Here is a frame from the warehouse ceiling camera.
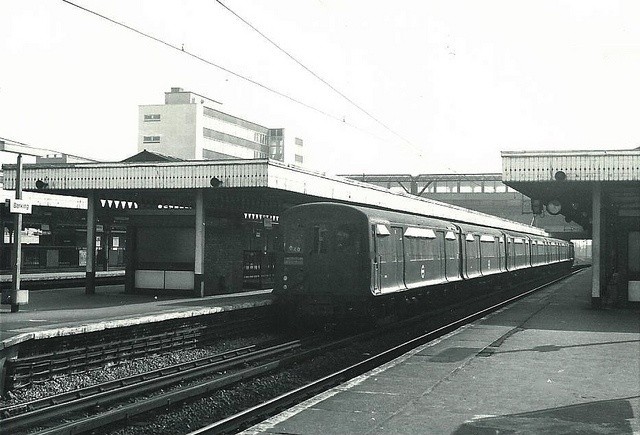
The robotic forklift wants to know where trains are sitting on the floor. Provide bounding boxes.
[271,201,575,324]
